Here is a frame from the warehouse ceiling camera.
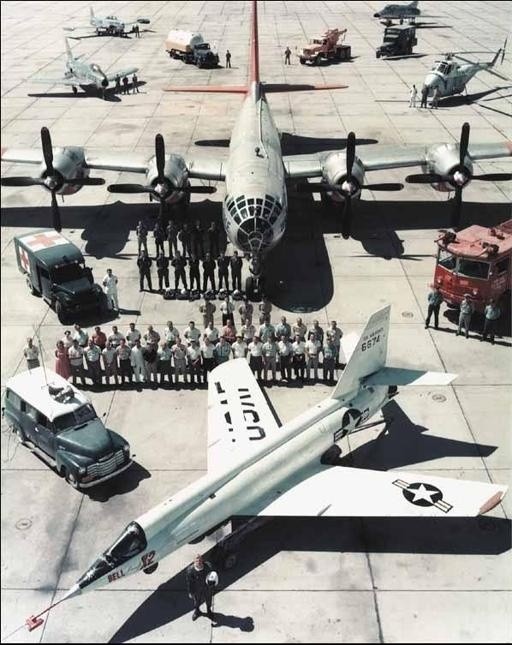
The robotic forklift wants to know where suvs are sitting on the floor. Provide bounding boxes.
[6,367,133,490]
[13,229,107,325]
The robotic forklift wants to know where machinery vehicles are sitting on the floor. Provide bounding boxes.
[298,28,346,66]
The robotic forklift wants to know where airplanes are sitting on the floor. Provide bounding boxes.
[3,303,510,640]
[2,2,512,285]
[24,37,141,99]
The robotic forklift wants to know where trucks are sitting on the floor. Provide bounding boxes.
[166,30,217,68]
[433,219,512,319]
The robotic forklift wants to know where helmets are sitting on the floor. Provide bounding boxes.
[204,570,221,587]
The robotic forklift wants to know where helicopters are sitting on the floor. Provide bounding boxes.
[373,1,451,28]
[374,39,507,103]
[64,3,153,40]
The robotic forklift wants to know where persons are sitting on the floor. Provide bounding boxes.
[409,85,417,108]
[238,298,253,327]
[132,74,139,93]
[116,339,133,385]
[276,335,293,384]
[186,553,214,620]
[260,296,272,327]
[184,321,201,346]
[74,323,89,346]
[142,341,158,388]
[136,221,148,257]
[292,318,307,342]
[291,334,306,382]
[97,25,140,39]
[231,337,248,359]
[431,85,440,109]
[154,222,165,258]
[123,75,129,94]
[166,220,178,260]
[200,337,217,384]
[305,334,322,381]
[189,253,200,293]
[114,74,121,95]
[137,251,154,292]
[62,332,74,349]
[157,342,173,387]
[24,338,40,370]
[247,337,264,384]
[173,338,188,387]
[84,342,101,388]
[261,338,280,385]
[217,251,230,293]
[200,296,216,330]
[226,50,231,67]
[91,328,106,349]
[215,338,231,364]
[164,322,179,348]
[222,319,236,344]
[284,47,291,65]
[480,298,501,343]
[143,326,161,352]
[156,252,169,292]
[101,341,119,388]
[192,220,206,260]
[126,323,141,347]
[103,269,120,313]
[131,340,146,385]
[68,340,87,387]
[308,321,323,367]
[325,321,343,367]
[241,319,256,344]
[420,85,429,108]
[220,297,235,326]
[322,336,338,386]
[55,340,70,380]
[425,285,443,330]
[179,223,192,258]
[457,294,475,338]
[275,317,292,341]
[207,223,221,260]
[258,318,275,343]
[231,252,243,293]
[203,322,219,345]
[185,340,202,387]
[108,327,126,347]
[171,251,188,291]
[202,253,216,293]
